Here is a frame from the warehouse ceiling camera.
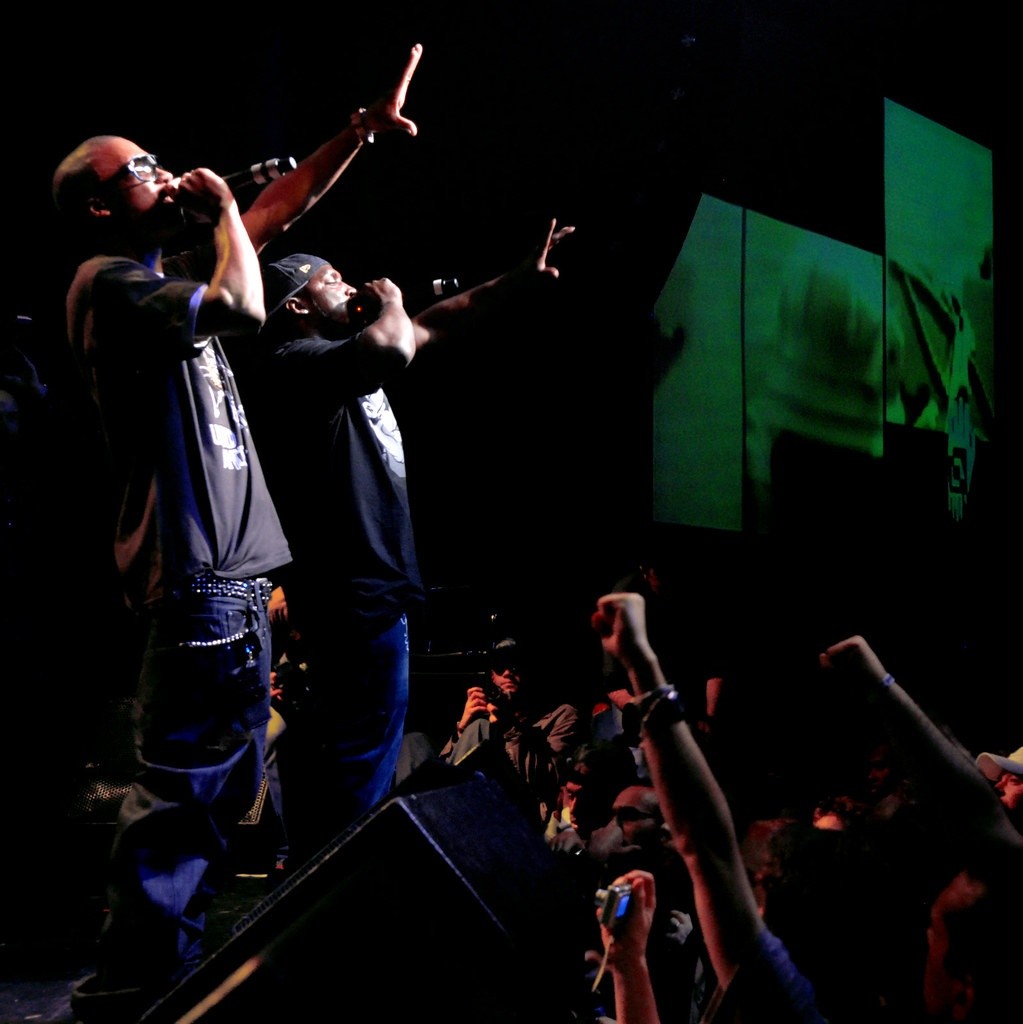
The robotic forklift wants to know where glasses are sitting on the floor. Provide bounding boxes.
[490,660,524,674]
[95,155,158,195]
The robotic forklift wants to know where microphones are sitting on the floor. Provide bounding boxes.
[221,157,296,190]
[401,279,460,303]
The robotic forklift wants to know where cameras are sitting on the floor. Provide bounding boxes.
[593,883,633,928]
[483,684,509,708]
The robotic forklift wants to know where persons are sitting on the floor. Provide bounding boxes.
[52,44,432,1024]
[257,219,594,880]
[585,589,1023,1024]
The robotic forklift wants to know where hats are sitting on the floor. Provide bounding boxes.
[974,745,1023,779]
[492,639,522,653]
[259,253,328,337]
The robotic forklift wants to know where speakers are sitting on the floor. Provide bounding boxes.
[138,774,598,1024]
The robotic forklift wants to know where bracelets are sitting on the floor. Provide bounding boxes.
[857,673,898,707]
[350,108,375,146]
[556,823,578,834]
[456,722,464,734]
[621,684,687,747]
